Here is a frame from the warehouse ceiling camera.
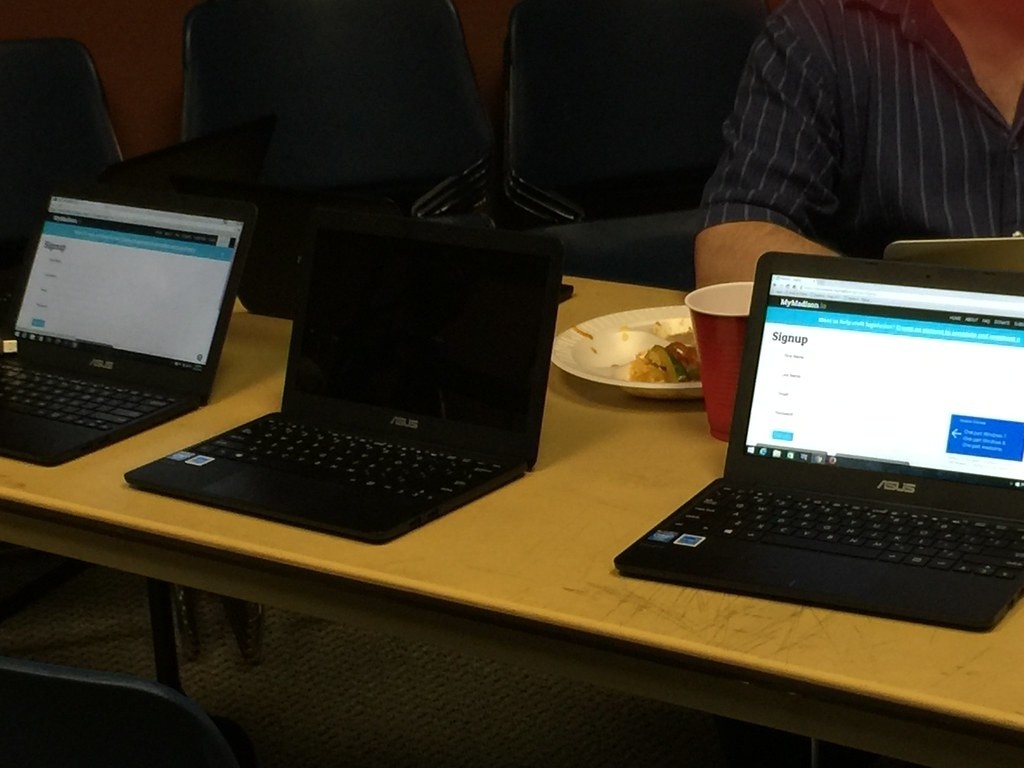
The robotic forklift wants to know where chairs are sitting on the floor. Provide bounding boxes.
[0,30,132,303]
[0,662,264,768]
[179,2,493,235]
[510,2,770,300]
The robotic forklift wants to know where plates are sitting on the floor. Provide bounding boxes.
[551,305,704,400]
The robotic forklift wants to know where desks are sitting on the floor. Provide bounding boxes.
[1,268,1016,733]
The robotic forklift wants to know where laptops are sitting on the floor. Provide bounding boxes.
[0,176,257,467]
[614,237,1024,634]
[128,207,567,547]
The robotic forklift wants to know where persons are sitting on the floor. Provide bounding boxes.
[695,0,1022,295]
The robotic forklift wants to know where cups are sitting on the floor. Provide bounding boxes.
[684,282,755,442]
